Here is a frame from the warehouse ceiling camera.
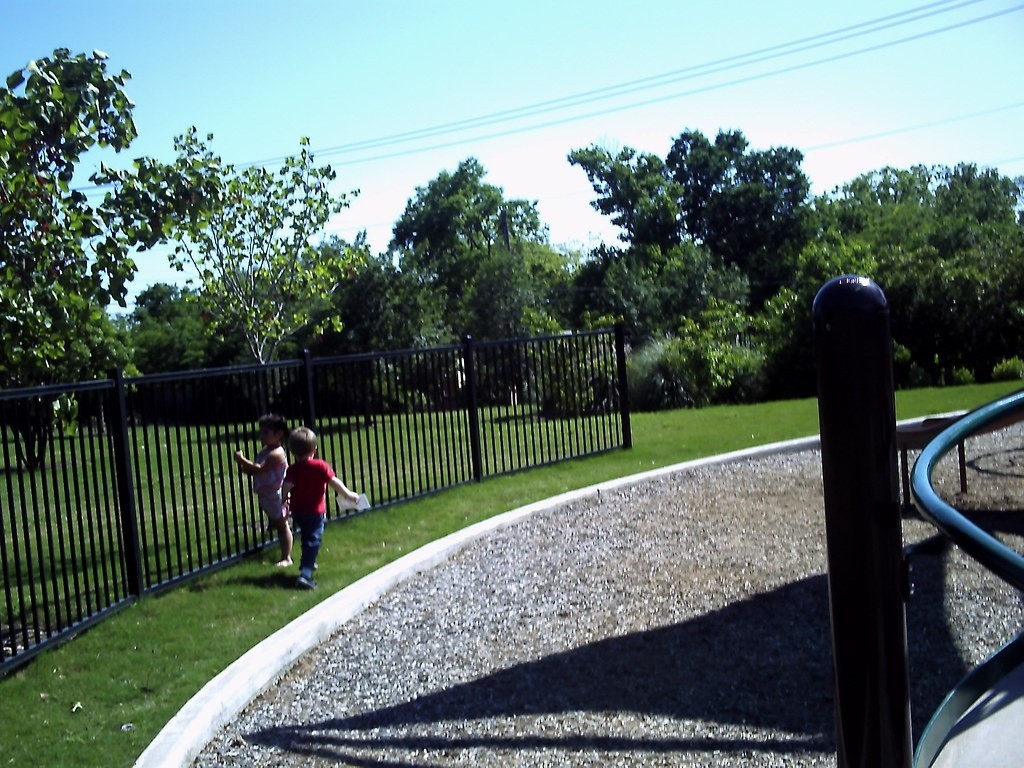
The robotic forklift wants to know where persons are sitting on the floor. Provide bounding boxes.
[234,415,295,568]
[280,426,360,589]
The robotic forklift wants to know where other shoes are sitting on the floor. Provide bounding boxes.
[298,564,318,570]
[275,560,293,568]
[298,575,315,589]
